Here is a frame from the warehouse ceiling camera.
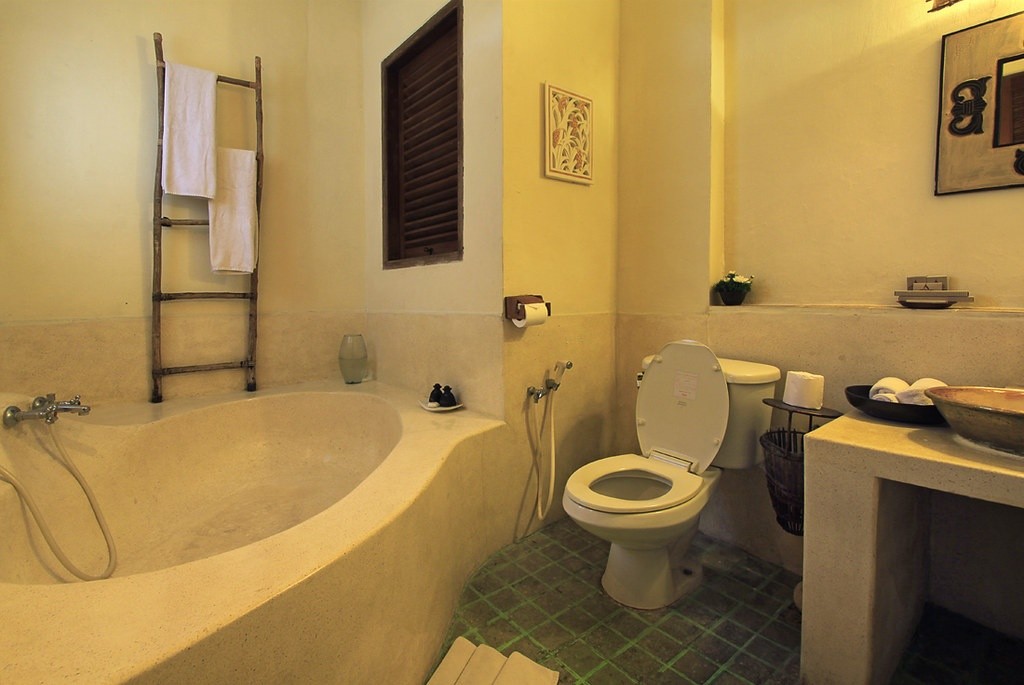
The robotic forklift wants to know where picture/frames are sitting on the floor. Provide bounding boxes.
[933,10,1024,196]
[546,80,596,186]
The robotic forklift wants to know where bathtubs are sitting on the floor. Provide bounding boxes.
[0,388,407,590]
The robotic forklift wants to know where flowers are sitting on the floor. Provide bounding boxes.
[714,269,754,293]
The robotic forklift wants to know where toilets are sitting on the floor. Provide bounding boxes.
[560,339,780,608]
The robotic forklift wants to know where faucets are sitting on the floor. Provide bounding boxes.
[31,394,91,419]
[3,392,58,427]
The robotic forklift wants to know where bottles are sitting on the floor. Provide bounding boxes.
[336,332,368,386]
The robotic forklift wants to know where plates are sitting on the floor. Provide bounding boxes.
[420,400,464,412]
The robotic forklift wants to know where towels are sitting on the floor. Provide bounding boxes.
[899,378,948,405]
[162,61,218,200]
[208,147,259,275]
[872,392,899,404]
[870,376,908,395]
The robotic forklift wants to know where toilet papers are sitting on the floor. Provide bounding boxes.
[509,304,548,328]
[785,371,825,410]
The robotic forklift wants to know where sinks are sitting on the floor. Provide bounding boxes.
[924,385,1024,457]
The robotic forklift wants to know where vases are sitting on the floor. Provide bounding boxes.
[720,289,746,306]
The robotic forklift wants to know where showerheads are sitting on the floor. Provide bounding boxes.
[525,361,576,519]
